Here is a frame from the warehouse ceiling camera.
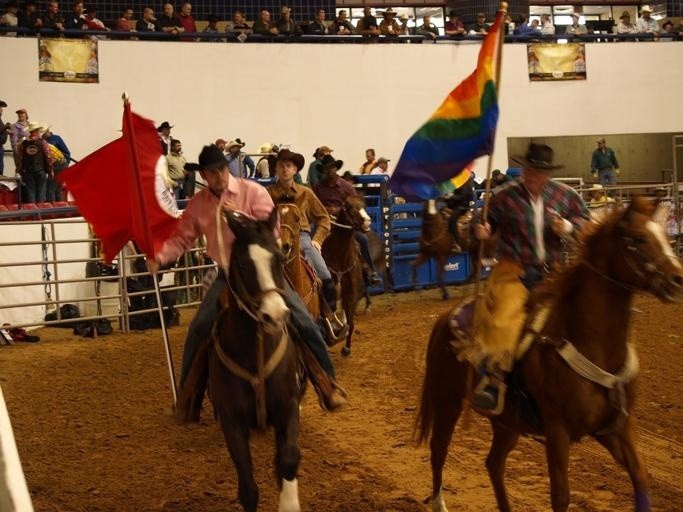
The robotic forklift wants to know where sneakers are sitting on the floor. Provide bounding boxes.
[471,383,498,408]
[323,390,348,410]
[450,244,462,251]
[369,271,383,286]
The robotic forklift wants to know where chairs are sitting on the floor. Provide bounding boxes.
[0,192,77,221]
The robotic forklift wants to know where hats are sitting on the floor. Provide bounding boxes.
[378,158,390,164]
[509,144,565,169]
[183,138,343,175]
[360,6,580,18]
[0,100,49,135]
[620,5,673,29]
[596,137,605,144]
[157,122,175,132]
[280,7,292,14]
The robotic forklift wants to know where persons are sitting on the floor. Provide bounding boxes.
[157,122,278,209]
[459,143,590,413]
[145,144,347,417]
[308,146,390,188]
[1,100,70,203]
[311,154,382,284]
[469,169,513,200]
[446,171,475,251]
[265,148,337,318]
[591,137,618,198]
[590,184,614,202]
[1,1,682,43]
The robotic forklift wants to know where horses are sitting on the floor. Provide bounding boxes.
[206,205,307,512]
[269,190,320,412]
[411,193,683,512]
[410,199,482,301]
[321,191,385,357]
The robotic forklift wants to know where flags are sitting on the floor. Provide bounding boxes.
[55,111,179,265]
[386,15,504,202]
